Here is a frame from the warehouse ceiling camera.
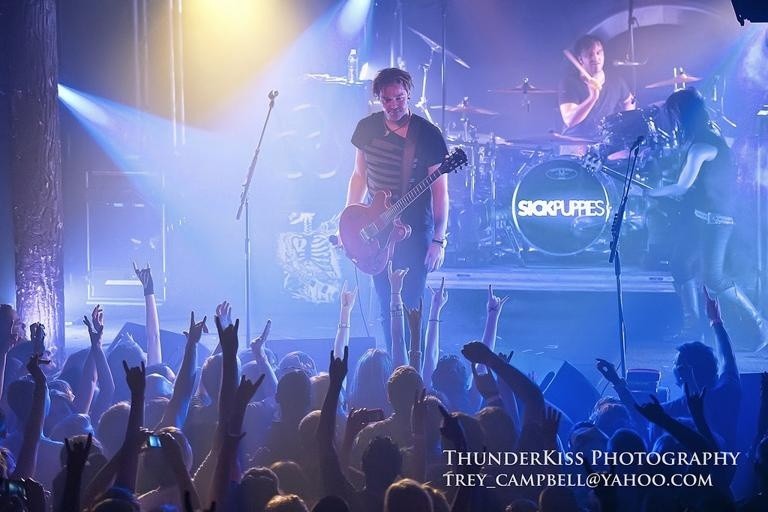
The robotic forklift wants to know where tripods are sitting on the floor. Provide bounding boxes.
[470,151,525,271]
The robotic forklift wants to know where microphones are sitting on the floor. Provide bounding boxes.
[631,135,647,151]
[268,88,280,100]
[613,60,645,70]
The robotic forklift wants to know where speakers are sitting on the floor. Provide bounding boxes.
[253,336,376,388]
[83,166,170,309]
[106,322,216,391]
[538,359,603,426]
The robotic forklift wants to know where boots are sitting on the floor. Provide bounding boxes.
[668,276,707,348]
[715,280,768,355]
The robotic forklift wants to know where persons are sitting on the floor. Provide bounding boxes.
[0,258,768,512]
[623,84,767,357]
[328,67,450,359]
[554,33,638,165]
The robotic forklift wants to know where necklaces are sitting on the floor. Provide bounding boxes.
[384,108,411,139]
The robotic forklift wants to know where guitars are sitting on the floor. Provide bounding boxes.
[580,153,688,214]
[339,147,469,276]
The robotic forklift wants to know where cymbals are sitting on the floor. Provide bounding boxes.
[504,133,597,145]
[448,104,498,115]
[647,100,666,109]
[487,83,567,95]
[645,74,704,90]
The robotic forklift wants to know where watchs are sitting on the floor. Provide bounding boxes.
[431,238,448,248]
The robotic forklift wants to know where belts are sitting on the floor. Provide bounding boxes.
[693,207,734,226]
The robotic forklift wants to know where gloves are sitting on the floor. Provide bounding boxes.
[134,267,155,297]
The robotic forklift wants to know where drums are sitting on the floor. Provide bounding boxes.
[597,107,658,160]
[446,131,505,193]
[512,155,613,257]
